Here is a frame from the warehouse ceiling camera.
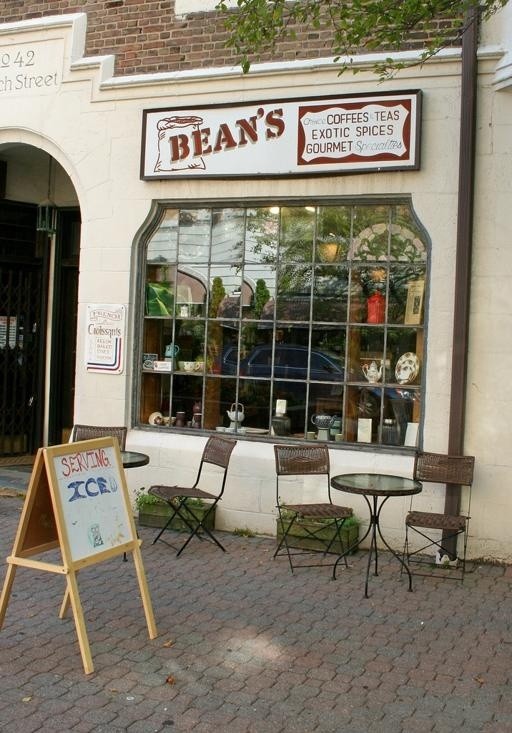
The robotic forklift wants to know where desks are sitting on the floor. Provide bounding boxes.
[328,472,422,601]
[115,442,149,564]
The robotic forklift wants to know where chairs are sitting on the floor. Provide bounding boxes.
[69,423,128,451]
[401,451,476,586]
[151,433,238,556]
[272,444,350,577]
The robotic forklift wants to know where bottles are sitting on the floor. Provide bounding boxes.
[383,418,397,444]
[367,288,385,325]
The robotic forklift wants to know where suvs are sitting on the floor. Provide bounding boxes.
[221,345,397,419]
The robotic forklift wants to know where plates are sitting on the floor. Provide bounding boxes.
[246,427,270,434]
[148,412,163,426]
[394,351,420,385]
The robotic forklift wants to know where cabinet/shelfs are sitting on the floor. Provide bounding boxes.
[140,264,426,447]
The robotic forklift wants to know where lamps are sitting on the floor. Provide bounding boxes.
[36,154,58,240]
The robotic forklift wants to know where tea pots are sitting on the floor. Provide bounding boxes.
[226,402,245,422]
[361,360,383,383]
[164,343,180,357]
[310,412,338,429]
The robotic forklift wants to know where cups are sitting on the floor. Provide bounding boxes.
[306,431,316,439]
[165,412,202,428]
[181,307,189,318]
[216,427,246,435]
[178,361,204,372]
[334,434,345,442]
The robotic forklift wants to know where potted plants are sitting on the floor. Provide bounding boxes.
[276,503,362,557]
[134,488,216,536]
